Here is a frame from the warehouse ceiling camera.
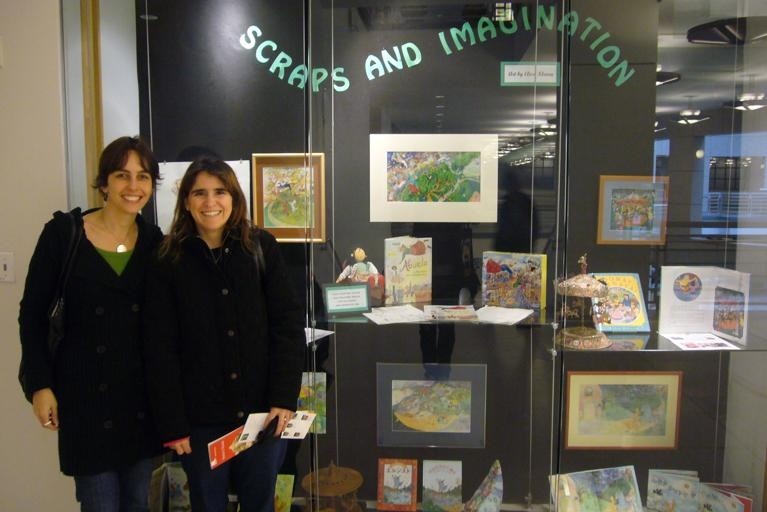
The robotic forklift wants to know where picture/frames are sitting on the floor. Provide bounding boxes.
[250,152,325,245]
[563,369,683,452]
[422,460,462,506]
[377,459,418,512]
[367,131,499,226]
[373,360,487,452]
[597,175,670,248]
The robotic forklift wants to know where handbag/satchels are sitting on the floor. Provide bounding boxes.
[43,209,85,380]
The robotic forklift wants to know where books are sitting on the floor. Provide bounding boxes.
[383,235,432,303]
[645,468,700,512]
[701,482,756,512]
[478,251,546,310]
[588,271,651,334]
[658,265,751,346]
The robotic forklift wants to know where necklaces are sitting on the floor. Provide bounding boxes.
[102,209,137,254]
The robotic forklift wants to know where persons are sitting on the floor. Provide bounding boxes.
[412,153,469,390]
[136,158,307,510]
[489,154,541,311]
[16,133,165,512]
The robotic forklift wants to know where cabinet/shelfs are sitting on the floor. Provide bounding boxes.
[309,0,564,511]
[551,1,767,512]
[83,0,317,512]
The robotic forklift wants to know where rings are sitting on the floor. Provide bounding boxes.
[43,420,52,427]
